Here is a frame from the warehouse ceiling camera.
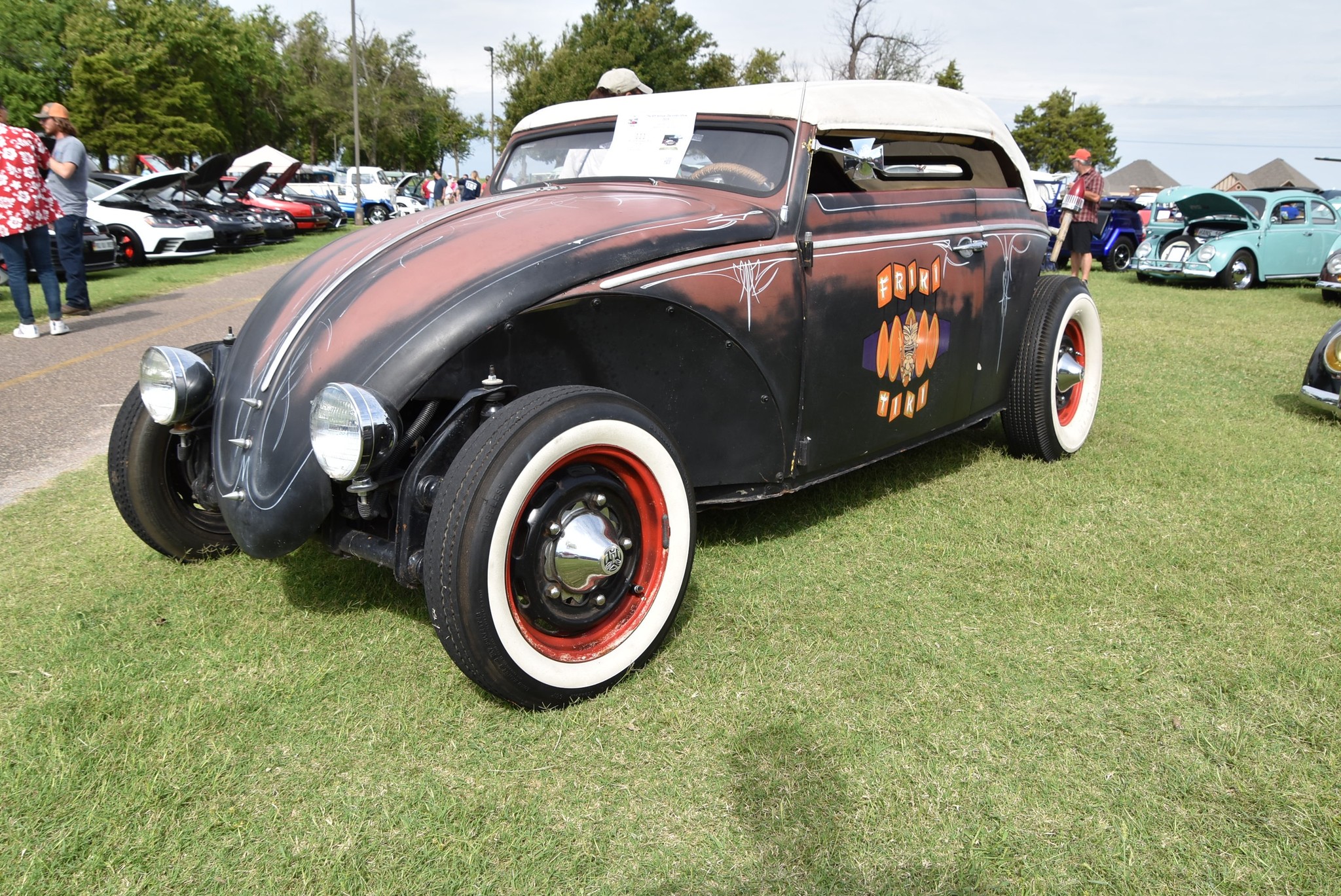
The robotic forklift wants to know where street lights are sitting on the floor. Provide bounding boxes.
[484,45,494,173]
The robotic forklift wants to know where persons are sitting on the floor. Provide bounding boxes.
[420,169,490,210]
[38,104,91,315]
[501,173,543,191]
[588,66,653,100]
[1280,202,1300,220]
[0,102,71,338]
[1059,148,1104,283]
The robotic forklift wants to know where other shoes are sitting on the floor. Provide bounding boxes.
[49,320,69,335]
[13,323,40,338]
[61,304,89,316]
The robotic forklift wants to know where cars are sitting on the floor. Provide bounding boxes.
[1033,176,1341,420]
[0,154,428,283]
[106,78,1101,711]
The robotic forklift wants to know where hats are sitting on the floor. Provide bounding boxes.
[597,67,653,94]
[1068,149,1091,161]
[31,102,70,119]
[448,173,452,176]
